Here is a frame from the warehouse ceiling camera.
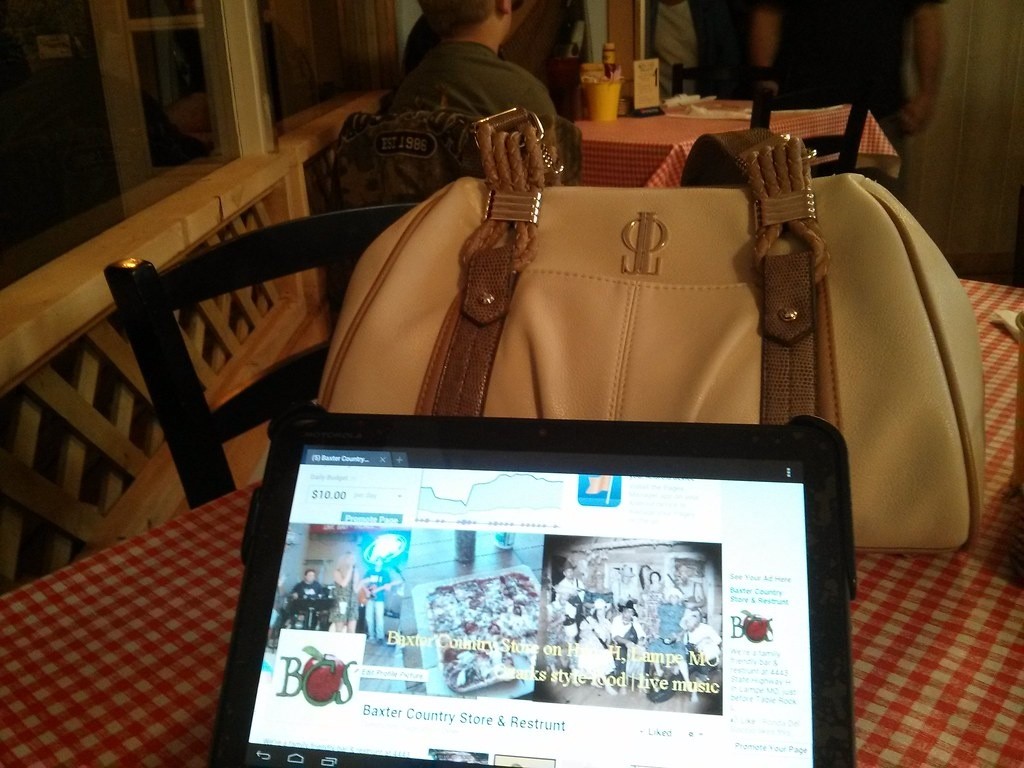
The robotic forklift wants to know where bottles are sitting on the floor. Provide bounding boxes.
[603,42,616,79]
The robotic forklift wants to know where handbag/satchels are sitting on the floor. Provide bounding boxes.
[314,108,986,557]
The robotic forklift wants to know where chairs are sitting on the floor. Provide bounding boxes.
[752,78,871,178]
[102,203,421,512]
[327,107,583,226]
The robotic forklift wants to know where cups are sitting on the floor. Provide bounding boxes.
[455,530,476,562]
[584,80,622,122]
[495,531,516,549]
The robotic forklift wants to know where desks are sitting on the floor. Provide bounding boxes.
[0,276,1024,768]
[574,100,901,189]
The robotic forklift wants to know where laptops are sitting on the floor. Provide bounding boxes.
[217,410,855,768]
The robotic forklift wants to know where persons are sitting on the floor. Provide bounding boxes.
[546,564,722,712]
[650,0,949,194]
[390,0,558,187]
[287,556,393,644]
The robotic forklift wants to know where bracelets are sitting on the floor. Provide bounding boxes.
[748,66,778,84]
